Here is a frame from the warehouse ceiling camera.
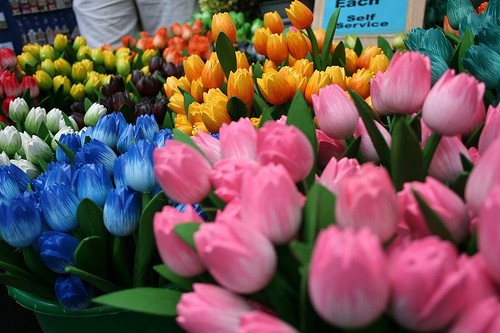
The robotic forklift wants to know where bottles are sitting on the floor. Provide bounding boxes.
[10,0,56,16]
[16,17,72,47]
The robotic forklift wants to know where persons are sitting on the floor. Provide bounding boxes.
[73,0,196,51]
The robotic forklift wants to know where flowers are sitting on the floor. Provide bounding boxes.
[0,0,500,333]
